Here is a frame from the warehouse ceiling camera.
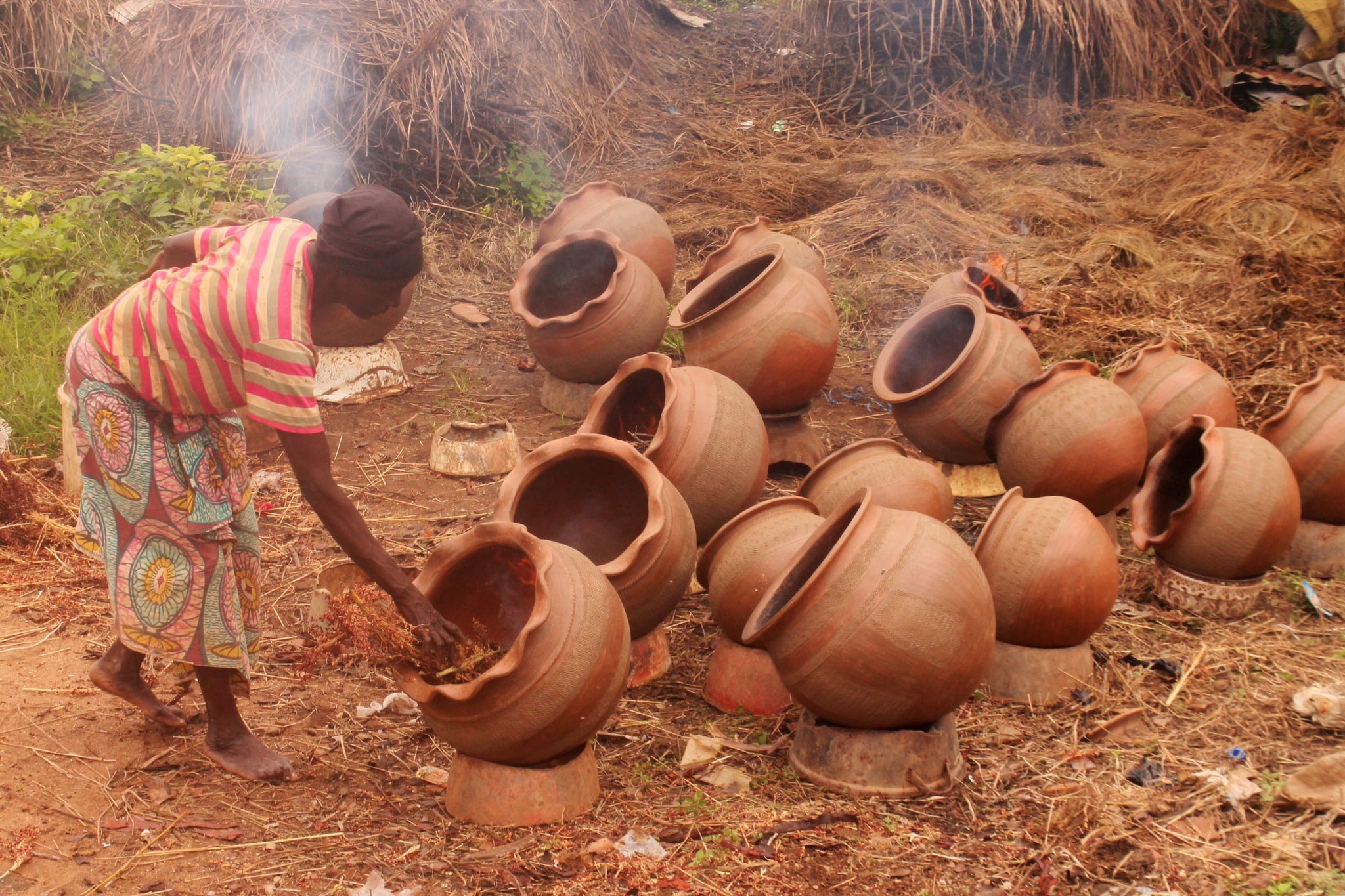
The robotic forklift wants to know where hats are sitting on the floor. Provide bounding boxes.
[317,184,426,280]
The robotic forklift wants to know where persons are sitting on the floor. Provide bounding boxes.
[52,176,466,784]
